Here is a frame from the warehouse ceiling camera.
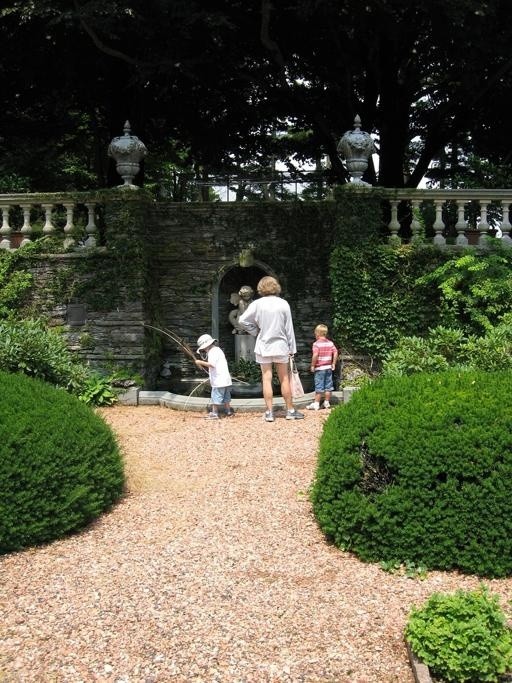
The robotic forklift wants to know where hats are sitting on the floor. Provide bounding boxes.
[196,333,219,355]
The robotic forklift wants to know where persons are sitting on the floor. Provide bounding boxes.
[238,285,258,333]
[228,291,243,332]
[193,332,235,422]
[305,321,339,410]
[236,274,306,421]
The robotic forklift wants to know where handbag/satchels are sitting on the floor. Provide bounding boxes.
[288,355,305,399]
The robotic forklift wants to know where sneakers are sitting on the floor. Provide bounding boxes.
[203,408,234,420]
[285,408,304,420]
[305,402,320,411]
[320,402,331,409]
[262,409,275,422]
